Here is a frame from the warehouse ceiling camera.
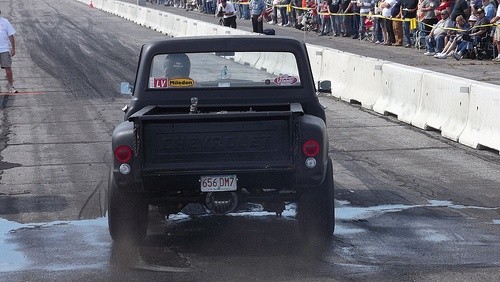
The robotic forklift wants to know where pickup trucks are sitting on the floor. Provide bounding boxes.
[107,34,336,249]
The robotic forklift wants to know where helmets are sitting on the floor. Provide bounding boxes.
[163,54,191,76]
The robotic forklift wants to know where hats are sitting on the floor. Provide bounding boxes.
[469,15,477,21]
[476,8,484,13]
[441,10,447,14]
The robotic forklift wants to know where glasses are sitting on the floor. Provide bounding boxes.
[477,13,481,14]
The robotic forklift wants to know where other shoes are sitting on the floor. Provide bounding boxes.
[492,56,500,62]
[334,33,340,37]
[405,44,410,48]
[424,51,434,55]
[434,52,439,57]
[352,34,358,39]
[447,51,454,57]
[452,52,460,60]
[8,87,18,93]
[375,41,402,46]
[438,52,447,59]
[343,34,350,37]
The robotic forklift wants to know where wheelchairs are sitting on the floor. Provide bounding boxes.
[462,28,498,60]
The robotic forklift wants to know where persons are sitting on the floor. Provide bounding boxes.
[235,0,250,20]
[266,0,500,62]
[147,0,217,15]
[215,0,237,29]
[250,0,266,34]
[163,52,201,88]
[0,10,19,93]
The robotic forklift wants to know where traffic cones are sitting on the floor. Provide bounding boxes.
[89,0,93,8]
